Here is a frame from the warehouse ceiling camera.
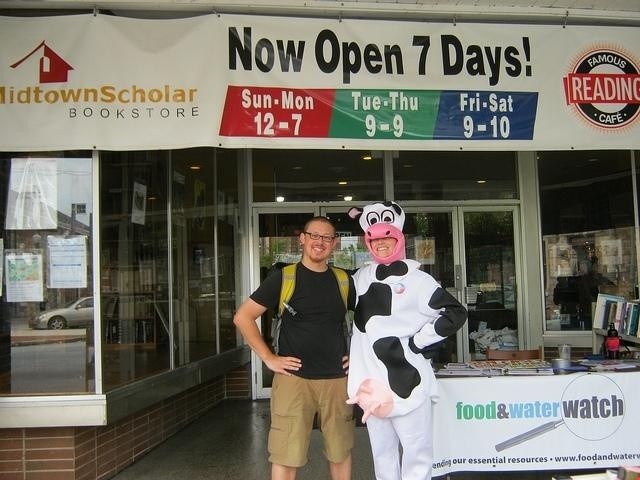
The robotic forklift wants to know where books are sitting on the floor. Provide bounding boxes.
[438,359,553,375]
[593,292,640,337]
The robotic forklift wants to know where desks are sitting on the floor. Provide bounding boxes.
[431,357,639,478]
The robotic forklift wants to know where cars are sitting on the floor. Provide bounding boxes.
[29,296,93,330]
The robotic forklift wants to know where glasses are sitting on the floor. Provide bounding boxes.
[304,231,335,242]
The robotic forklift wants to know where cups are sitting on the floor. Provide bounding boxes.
[559,344,571,365]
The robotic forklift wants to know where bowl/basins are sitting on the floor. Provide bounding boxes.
[550,357,570,369]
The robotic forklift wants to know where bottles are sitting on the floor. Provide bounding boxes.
[605,322,620,361]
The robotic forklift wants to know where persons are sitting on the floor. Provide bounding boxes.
[553,276,591,330]
[233,216,356,480]
[345,237,468,480]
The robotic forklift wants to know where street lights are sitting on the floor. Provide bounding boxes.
[33,232,46,312]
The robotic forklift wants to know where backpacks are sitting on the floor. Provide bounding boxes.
[271,264,352,354]
